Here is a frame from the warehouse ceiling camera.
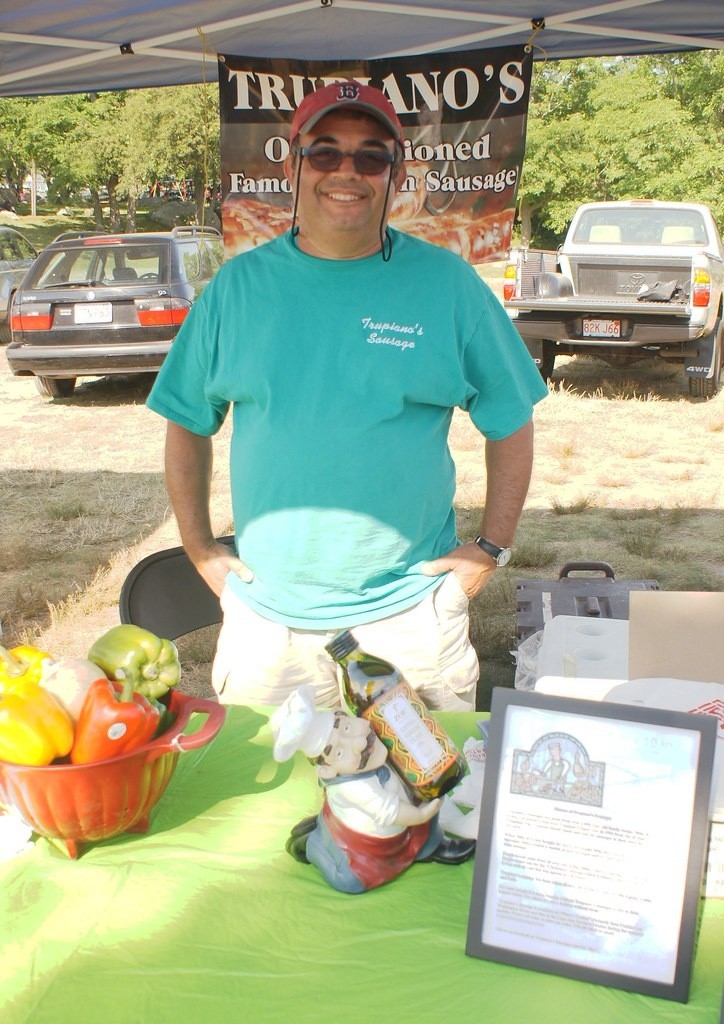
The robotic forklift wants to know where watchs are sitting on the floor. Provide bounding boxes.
[474,535,512,567]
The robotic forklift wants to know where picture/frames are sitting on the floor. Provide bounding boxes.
[463,688,718,1005]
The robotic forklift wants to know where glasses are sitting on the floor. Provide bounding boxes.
[300,145,395,176]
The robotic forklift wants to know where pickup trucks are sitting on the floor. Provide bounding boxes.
[503,198,724,396]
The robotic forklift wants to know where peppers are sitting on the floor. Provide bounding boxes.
[0,624,181,767]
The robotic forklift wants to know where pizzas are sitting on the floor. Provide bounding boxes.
[221,197,299,259]
[384,158,515,263]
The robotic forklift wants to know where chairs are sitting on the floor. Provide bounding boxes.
[112,266,139,282]
[118,534,237,642]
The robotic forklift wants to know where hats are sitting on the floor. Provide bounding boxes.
[289,81,405,159]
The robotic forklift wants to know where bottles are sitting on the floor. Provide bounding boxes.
[322,628,467,801]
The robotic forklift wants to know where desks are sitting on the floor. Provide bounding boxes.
[0,702,722,1024]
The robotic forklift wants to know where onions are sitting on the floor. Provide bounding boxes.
[39,657,108,722]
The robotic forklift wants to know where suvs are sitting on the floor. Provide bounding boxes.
[5,224,224,400]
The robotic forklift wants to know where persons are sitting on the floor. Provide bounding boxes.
[270,683,477,894]
[143,81,548,712]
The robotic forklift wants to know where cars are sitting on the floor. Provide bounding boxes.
[140,180,180,199]
[181,179,196,199]
[0,227,41,342]
[86,185,128,203]
[20,186,45,205]
[0,187,18,210]
